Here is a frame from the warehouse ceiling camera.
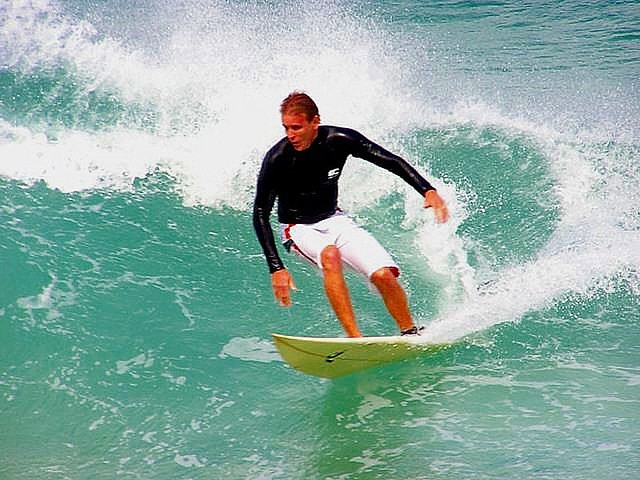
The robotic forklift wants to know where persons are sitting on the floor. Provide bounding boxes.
[252,90,448,336]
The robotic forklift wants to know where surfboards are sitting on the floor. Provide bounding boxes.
[271,333,455,378]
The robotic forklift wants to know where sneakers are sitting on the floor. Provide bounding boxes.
[401,326,424,336]
